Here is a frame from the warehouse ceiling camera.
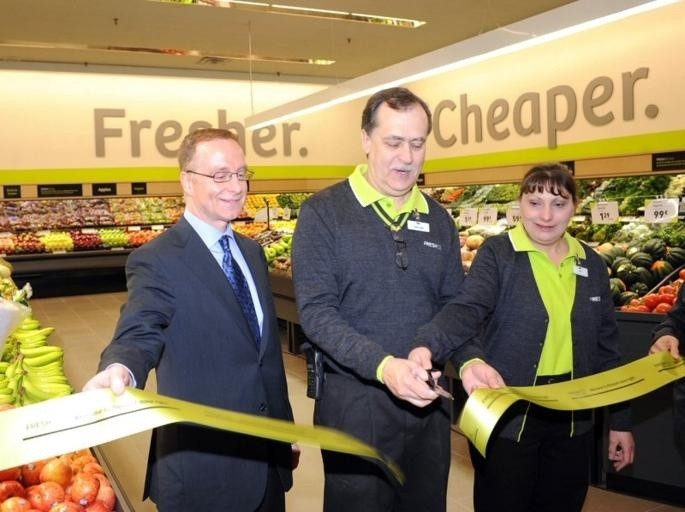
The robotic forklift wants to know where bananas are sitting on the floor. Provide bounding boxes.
[0,311,75,407]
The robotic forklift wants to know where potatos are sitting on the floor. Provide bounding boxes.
[460,235,485,272]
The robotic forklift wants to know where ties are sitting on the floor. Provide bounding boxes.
[217,237,262,350]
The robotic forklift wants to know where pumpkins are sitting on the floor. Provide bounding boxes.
[597,239,685,308]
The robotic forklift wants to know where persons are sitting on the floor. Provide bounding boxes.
[80,127,302,511]
[649,278,685,461]
[291,88,508,510]
[407,166,637,511]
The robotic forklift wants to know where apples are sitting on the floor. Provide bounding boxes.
[0,403,116,512]
[264,234,292,269]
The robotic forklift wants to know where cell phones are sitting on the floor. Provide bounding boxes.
[306,347,324,400]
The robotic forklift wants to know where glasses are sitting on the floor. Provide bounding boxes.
[185,168,255,184]
